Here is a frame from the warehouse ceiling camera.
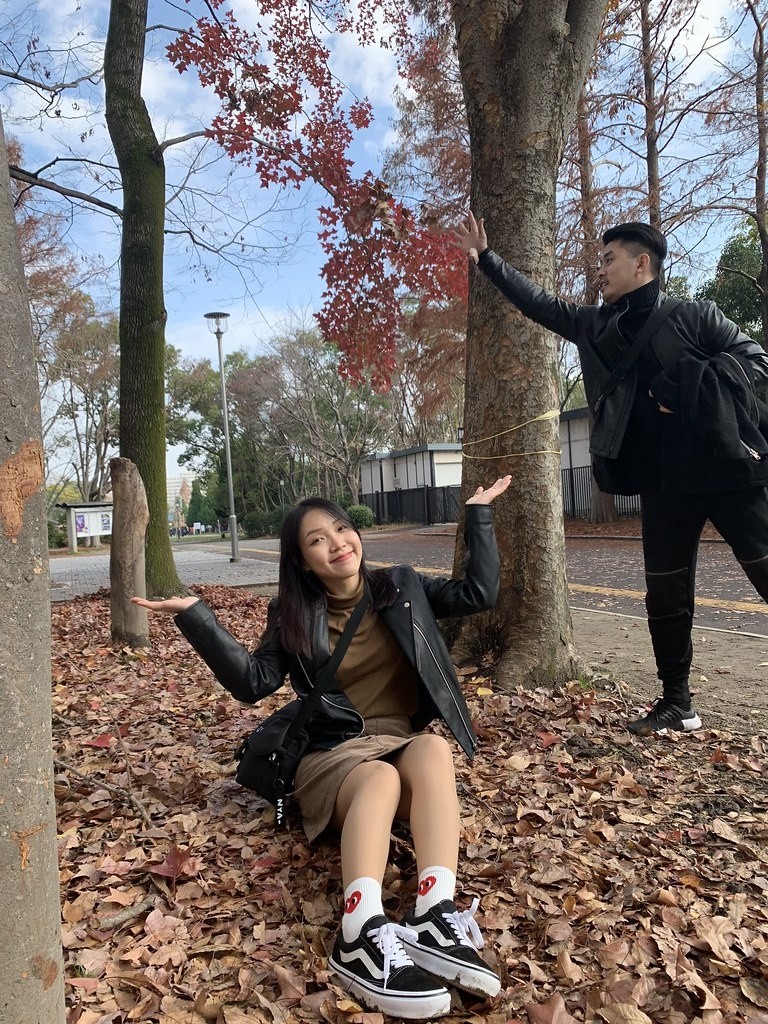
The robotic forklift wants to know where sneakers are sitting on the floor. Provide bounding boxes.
[397,898,501,998]
[628,697,703,738]
[328,914,452,1020]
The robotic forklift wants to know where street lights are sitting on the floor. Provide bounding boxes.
[204,311,239,562]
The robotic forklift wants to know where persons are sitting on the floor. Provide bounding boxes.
[448,210,768,736]
[130,475,512,1019]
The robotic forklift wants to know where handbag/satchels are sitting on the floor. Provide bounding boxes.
[233,697,317,831]
